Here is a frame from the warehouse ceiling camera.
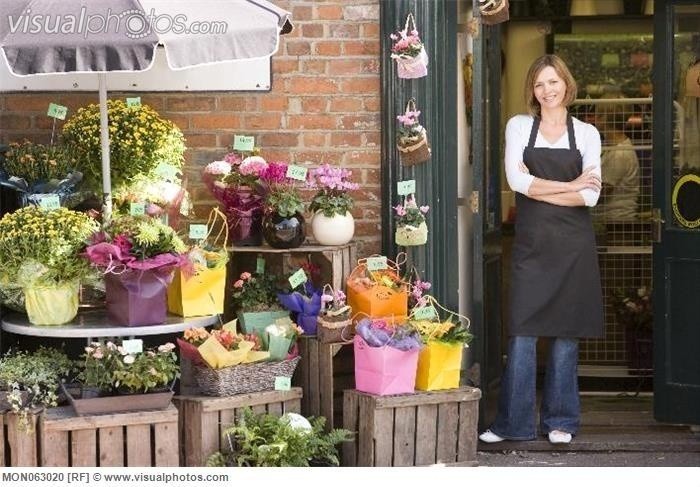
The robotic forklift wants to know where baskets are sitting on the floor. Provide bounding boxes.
[190,356,302,397]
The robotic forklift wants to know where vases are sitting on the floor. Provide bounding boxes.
[236,311,291,335]
[312,208,355,245]
[262,210,306,249]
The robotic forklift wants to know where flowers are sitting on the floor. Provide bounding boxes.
[234,273,285,312]
[354,319,420,351]
[396,110,425,146]
[204,151,270,201]
[279,264,328,300]
[320,283,347,312]
[80,214,190,269]
[350,265,407,294]
[394,198,430,230]
[181,240,229,269]
[259,316,305,362]
[183,318,270,369]
[4,139,87,195]
[304,163,361,217]
[63,100,188,203]
[0,207,102,290]
[410,314,474,344]
[389,30,422,60]
[411,280,432,308]
[73,341,181,394]
[259,162,304,217]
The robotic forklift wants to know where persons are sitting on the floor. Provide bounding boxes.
[476,54,603,446]
[621,66,685,194]
[589,90,642,268]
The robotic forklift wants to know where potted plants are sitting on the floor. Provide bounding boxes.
[206,407,358,467]
[1,345,72,436]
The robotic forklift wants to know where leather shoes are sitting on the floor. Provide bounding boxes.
[548,430,572,444]
[479,428,507,443]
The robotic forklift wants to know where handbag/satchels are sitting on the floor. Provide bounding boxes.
[316,284,351,344]
[395,185,428,247]
[396,13,429,79]
[165,208,229,316]
[480,0,510,26]
[408,296,471,392]
[396,100,432,167]
[96,243,179,327]
[348,252,408,334]
[342,312,420,396]
